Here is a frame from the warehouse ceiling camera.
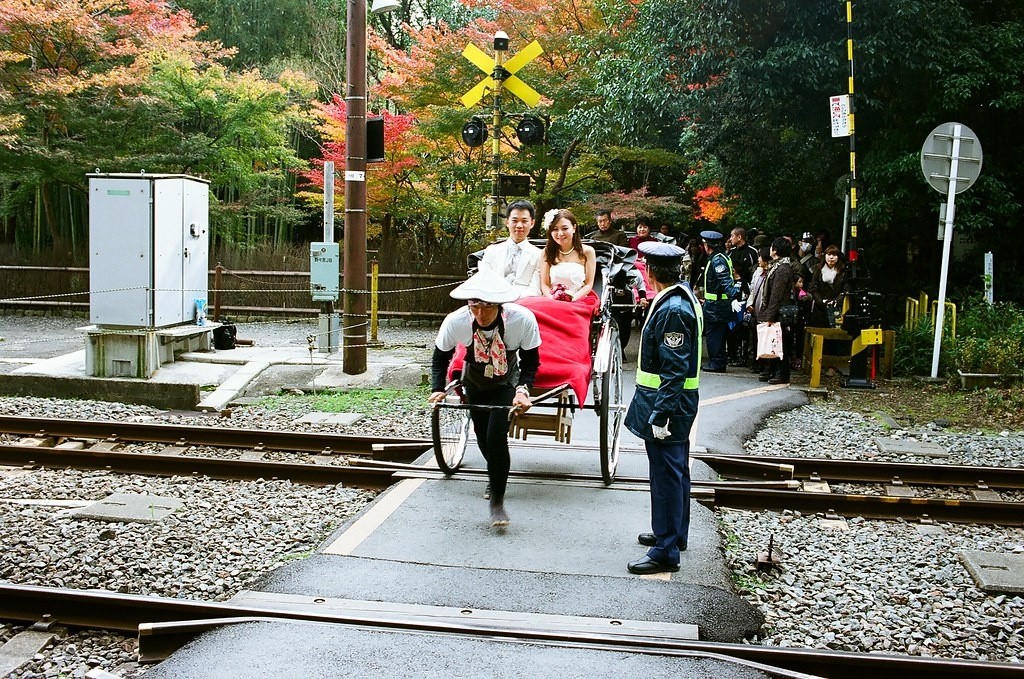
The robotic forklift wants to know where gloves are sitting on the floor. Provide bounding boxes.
[652,417,672,440]
[731,299,742,313]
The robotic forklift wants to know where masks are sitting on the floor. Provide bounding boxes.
[800,242,812,252]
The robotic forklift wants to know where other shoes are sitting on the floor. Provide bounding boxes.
[484,482,491,498]
[490,499,511,528]
[729,358,774,376]
[759,375,790,384]
[702,364,726,373]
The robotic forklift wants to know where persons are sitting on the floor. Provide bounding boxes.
[621,241,705,574]
[589,210,845,383]
[536,207,594,302]
[428,268,542,526]
[478,199,548,296]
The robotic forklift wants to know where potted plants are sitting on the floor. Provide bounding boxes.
[948,335,1024,390]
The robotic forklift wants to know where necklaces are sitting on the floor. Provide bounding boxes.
[558,245,574,255]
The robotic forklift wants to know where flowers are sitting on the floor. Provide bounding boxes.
[550,283,573,302]
[542,209,558,230]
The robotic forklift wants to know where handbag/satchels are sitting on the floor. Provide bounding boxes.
[779,305,799,324]
[756,322,783,360]
[744,312,756,324]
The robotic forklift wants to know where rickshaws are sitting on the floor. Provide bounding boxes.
[586,231,676,323]
[432,237,628,484]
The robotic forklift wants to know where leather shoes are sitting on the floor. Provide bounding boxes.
[627,554,682,575]
[638,532,687,551]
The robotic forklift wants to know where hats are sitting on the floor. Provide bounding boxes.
[637,241,685,265]
[798,232,815,244]
[449,269,521,304]
[701,231,723,242]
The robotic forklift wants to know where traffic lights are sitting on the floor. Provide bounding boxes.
[463,121,488,147]
[517,118,545,145]
[501,176,530,197]
